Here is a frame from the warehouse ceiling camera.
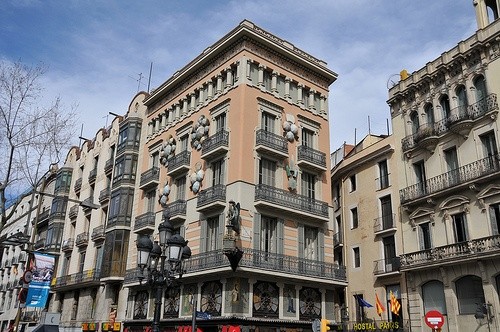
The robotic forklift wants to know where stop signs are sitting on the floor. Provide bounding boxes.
[424,310,444,330]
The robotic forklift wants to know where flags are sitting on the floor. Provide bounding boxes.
[390,290,401,316]
[375,293,386,317]
[25,251,56,309]
[357,294,374,308]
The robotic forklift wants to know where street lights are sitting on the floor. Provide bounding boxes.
[12,175,100,332]
[135,205,191,332]
